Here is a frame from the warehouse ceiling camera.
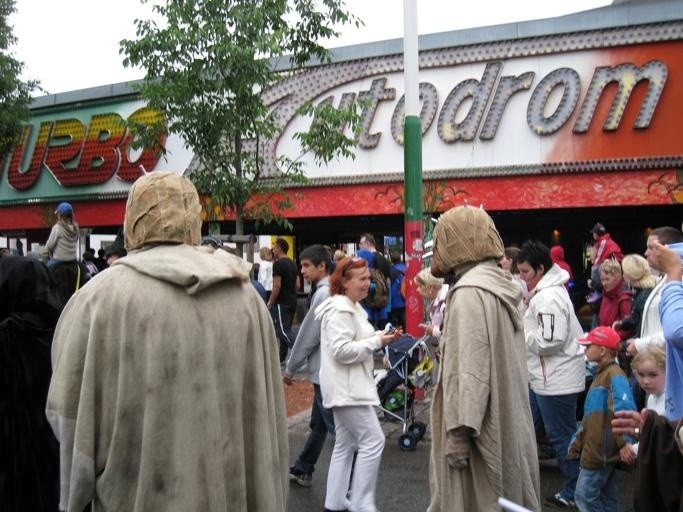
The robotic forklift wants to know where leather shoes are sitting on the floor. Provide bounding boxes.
[443,426,471,470]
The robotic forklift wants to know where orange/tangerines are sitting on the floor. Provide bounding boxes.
[373,320,433,453]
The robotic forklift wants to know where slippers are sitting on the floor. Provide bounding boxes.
[363,267,389,310]
[372,250,390,279]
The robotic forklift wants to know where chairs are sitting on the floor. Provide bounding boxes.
[279,361,286,370]
[537,446,558,463]
[289,464,313,487]
[543,492,578,510]
[295,356,308,373]
[586,290,602,304]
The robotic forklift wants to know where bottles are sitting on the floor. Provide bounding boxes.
[122,170,205,255]
[429,201,508,277]
[588,224,606,236]
[574,325,622,351]
[53,202,74,215]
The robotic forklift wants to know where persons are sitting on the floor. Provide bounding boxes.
[49,172,683,511]
